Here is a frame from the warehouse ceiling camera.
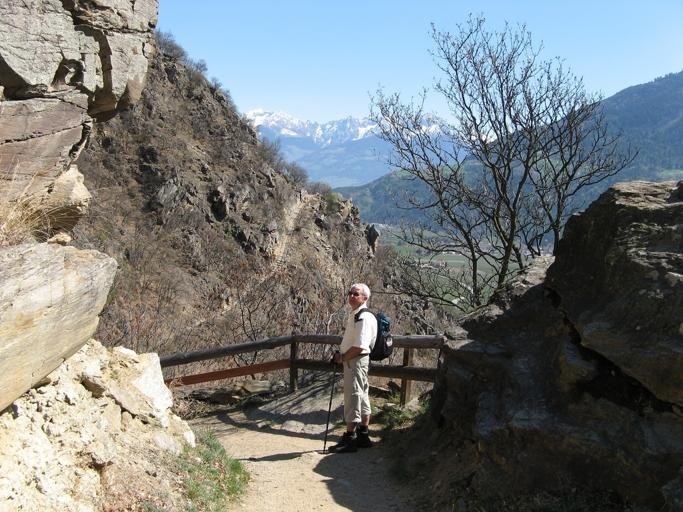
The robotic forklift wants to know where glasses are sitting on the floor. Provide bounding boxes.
[349,291,359,296]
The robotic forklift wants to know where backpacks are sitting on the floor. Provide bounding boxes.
[354,308,393,361]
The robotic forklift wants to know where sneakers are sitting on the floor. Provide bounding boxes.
[329,429,373,453]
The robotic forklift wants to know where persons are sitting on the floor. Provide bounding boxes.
[327,282,379,453]
